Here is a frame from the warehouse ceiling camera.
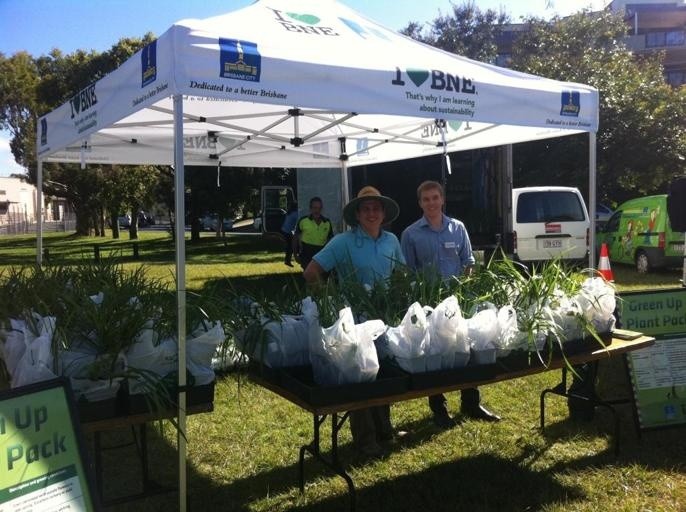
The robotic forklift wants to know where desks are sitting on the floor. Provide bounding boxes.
[76,372,214,506]
[248,311,656,512]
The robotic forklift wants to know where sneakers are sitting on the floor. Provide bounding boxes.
[285,261,294,267]
[360,435,406,461]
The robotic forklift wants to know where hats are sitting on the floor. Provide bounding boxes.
[343,186,401,226]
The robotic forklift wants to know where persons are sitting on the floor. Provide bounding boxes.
[280,201,297,268]
[302,187,406,456]
[292,196,334,272]
[401,181,501,427]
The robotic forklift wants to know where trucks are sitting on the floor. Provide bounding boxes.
[260,142,516,271]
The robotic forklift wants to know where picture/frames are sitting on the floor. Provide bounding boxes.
[614,287,685,433]
[0,377,102,512]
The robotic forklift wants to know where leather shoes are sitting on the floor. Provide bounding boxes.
[463,404,501,422]
[434,410,456,429]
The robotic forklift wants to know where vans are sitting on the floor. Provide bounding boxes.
[594,192,684,276]
[511,183,594,270]
